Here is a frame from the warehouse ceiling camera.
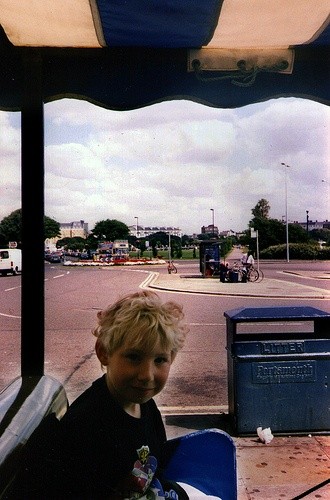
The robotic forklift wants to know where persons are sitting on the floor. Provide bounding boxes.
[0,291,190,500]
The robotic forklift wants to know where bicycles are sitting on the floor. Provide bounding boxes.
[225,267,264,283]
[166,260,177,274]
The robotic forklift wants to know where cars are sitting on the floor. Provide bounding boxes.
[50,255,62,263]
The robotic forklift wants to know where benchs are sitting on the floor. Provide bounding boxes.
[0,375,237,500]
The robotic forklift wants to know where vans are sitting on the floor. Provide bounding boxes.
[0,249,21,276]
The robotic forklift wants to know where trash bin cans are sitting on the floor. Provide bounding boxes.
[229,265,249,283]
[131,252,138,257]
[318,242,327,249]
[143,251,153,260]
[223,304,330,438]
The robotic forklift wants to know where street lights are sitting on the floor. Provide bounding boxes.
[281,162,291,263]
[134,216,139,231]
[209,208,215,233]
